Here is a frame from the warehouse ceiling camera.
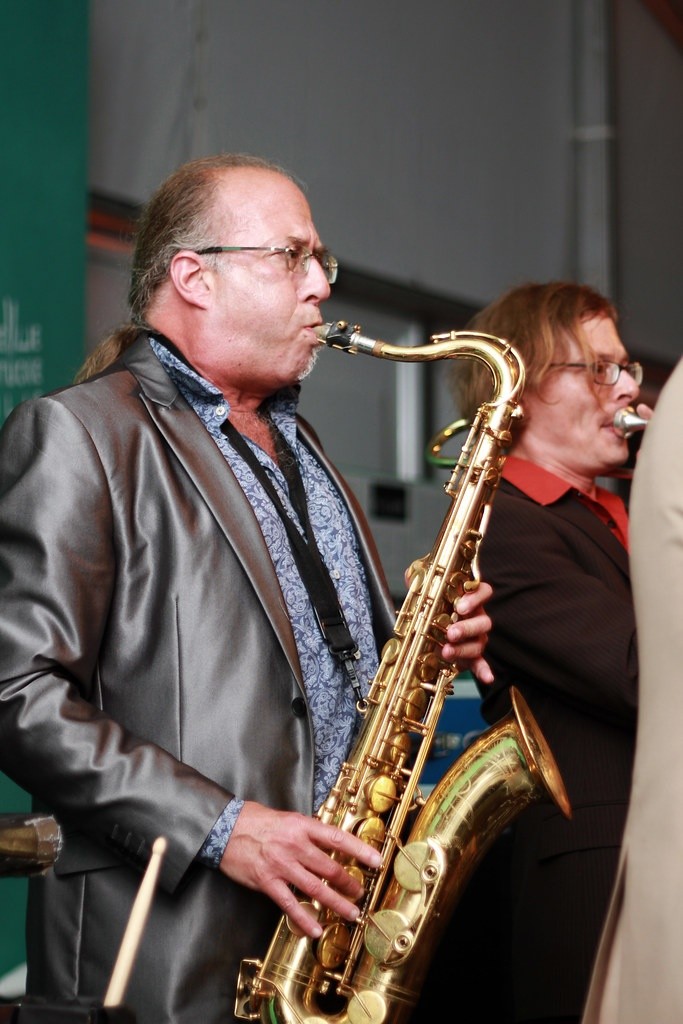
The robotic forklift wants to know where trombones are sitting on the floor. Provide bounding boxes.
[423,406,650,472]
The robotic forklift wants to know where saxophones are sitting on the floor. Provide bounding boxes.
[233,314,576,1024]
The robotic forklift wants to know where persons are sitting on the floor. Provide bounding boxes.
[444,278,683,1024]
[1,153,498,1024]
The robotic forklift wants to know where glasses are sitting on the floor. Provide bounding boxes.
[542,360,643,386]
[196,246,340,285]
